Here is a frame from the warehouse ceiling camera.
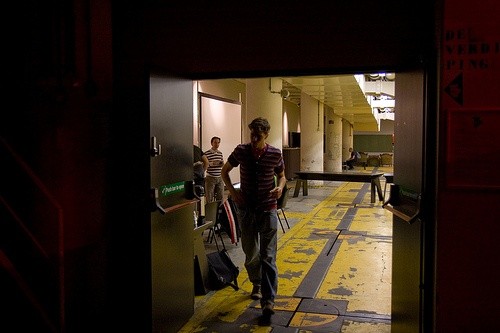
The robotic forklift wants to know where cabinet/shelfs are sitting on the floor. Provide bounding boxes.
[283,146,302,180]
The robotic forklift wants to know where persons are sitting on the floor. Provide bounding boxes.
[346,148,358,170]
[204,137,224,206]
[193,144,209,172]
[221,117,286,313]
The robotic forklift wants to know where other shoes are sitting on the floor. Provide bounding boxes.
[262,300,276,315]
[251,286,263,300]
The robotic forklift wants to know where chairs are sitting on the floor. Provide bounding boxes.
[277,182,290,233]
[378,153,394,167]
[204,196,240,250]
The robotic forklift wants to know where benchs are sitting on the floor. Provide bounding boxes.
[342,161,368,170]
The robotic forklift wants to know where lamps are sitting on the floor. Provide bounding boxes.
[279,88,291,101]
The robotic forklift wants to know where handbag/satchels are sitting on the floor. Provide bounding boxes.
[204,249,240,291]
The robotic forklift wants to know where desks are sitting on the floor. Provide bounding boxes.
[383,173,394,207]
[292,170,384,204]
